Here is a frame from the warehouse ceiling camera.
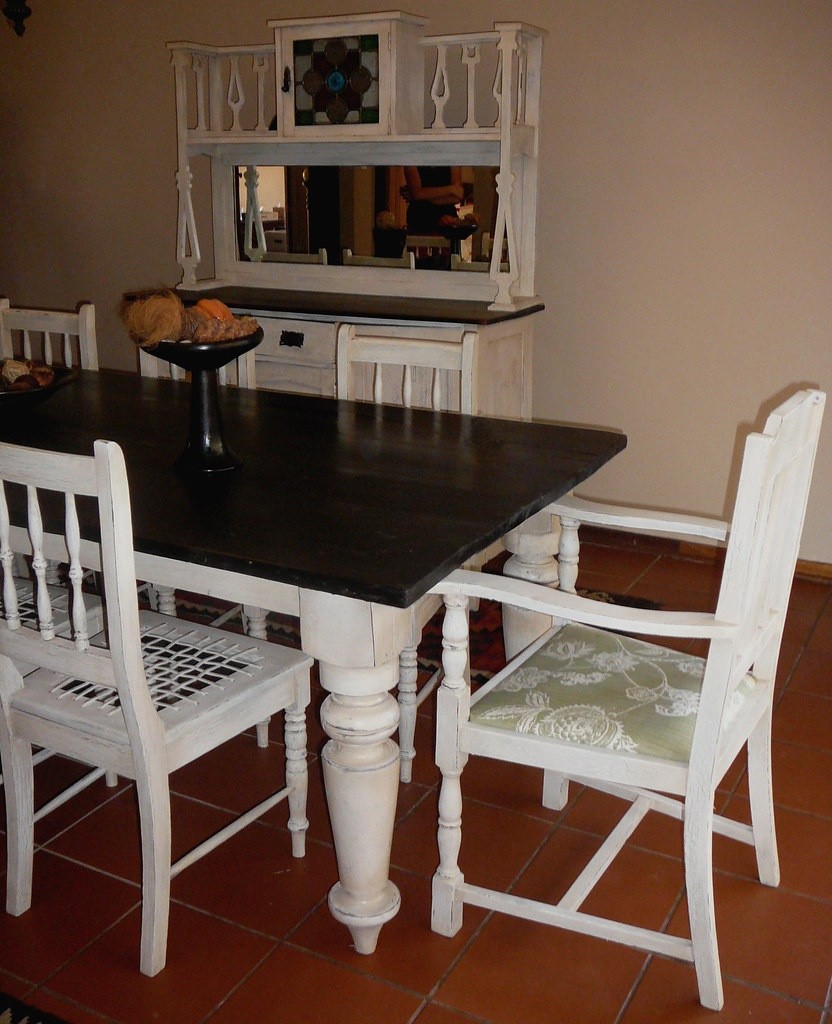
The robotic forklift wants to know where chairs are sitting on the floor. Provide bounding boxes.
[139,317,256,693]
[436,385,827,1010]
[254,322,480,779]
[1,296,101,587]
[0,439,317,983]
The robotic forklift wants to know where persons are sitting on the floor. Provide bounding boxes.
[399,166,464,233]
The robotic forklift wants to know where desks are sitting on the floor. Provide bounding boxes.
[0,367,629,954]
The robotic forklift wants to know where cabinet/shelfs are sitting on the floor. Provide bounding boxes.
[121,11,548,567]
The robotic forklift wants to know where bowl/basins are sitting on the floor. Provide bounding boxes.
[0,364,78,414]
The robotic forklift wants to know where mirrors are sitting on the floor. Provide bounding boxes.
[233,160,499,270]
